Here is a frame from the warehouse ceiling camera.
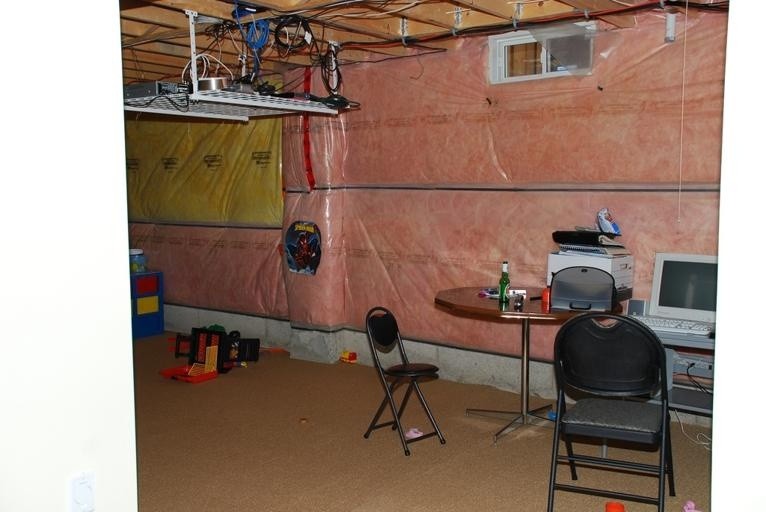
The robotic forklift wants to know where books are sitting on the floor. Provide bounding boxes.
[559,242,632,256]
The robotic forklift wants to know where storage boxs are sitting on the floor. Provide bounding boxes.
[130,272,164,338]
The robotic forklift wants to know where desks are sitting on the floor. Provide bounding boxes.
[626,329,715,415]
[435,287,623,442]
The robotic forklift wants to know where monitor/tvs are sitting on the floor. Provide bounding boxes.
[648,253,718,324]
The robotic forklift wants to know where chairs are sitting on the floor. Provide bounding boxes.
[547,311,675,512]
[364,306,445,455]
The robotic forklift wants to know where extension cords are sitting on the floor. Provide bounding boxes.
[678,358,713,370]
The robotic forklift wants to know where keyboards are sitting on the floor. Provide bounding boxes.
[630,317,712,335]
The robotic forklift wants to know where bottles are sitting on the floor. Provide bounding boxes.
[129,249,147,274]
[498,261,511,304]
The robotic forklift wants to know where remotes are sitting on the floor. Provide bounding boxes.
[514,295,523,307]
